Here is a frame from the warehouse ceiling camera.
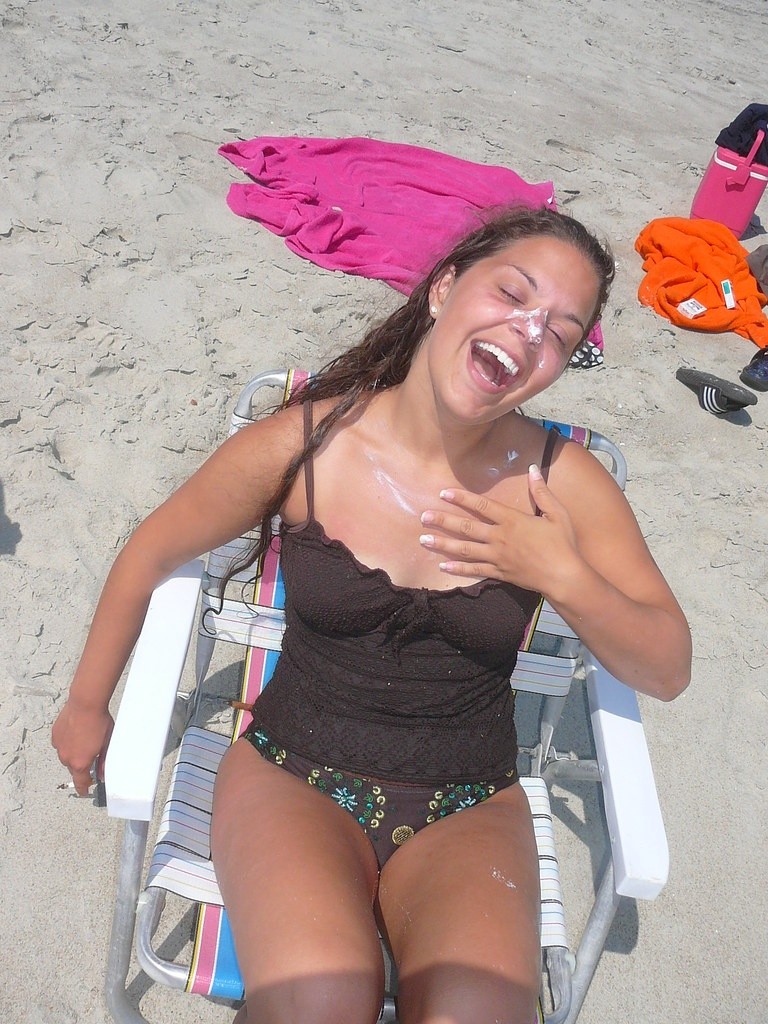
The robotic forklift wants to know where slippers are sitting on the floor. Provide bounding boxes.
[740,347,768,391]
[676,369,758,414]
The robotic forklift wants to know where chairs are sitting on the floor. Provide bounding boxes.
[106,369,671,1024]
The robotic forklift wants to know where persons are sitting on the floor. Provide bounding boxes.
[51,202,692,1024]
